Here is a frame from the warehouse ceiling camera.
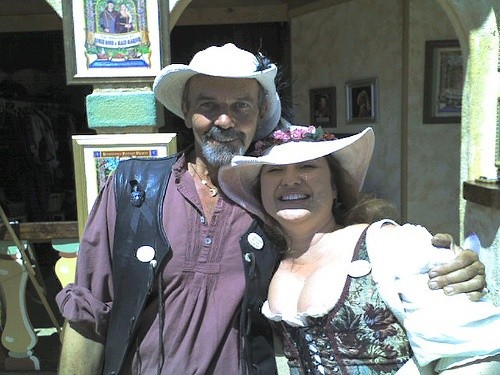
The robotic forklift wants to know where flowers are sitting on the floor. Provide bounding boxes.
[249,123,339,158]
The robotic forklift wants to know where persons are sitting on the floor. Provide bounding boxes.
[231,122,500,375]
[51,44,490,375]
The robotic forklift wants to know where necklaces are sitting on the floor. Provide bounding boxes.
[186,154,221,198]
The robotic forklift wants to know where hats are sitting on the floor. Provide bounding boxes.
[217,125,375,227]
[153,42,281,143]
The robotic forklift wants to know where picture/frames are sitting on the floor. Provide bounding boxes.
[343,77,380,125]
[64,0,172,86]
[422,39,465,126]
[309,87,336,129]
[72,134,178,243]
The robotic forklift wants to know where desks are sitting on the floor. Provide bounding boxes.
[0,220,80,371]
